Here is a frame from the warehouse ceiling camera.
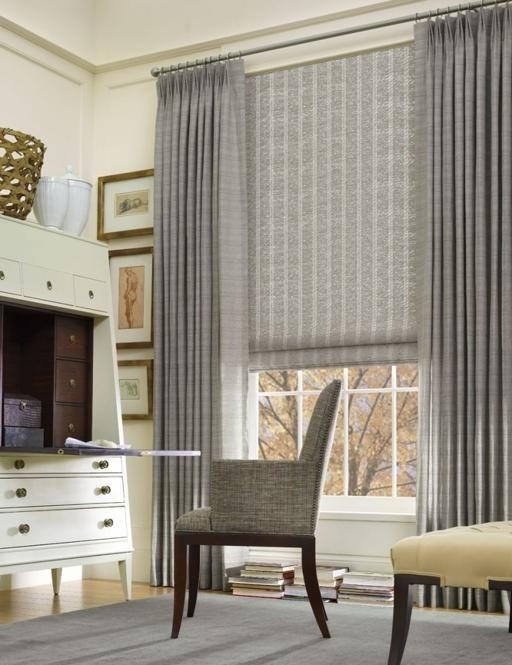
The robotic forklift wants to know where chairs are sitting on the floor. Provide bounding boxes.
[169,379,343,640]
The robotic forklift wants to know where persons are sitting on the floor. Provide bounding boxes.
[123,267,139,330]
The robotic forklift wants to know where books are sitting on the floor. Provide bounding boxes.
[228,561,395,607]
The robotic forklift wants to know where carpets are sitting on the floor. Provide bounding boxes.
[2,586,512,665]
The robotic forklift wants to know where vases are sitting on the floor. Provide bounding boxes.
[32,175,93,238]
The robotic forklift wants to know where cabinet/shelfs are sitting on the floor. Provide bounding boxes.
[1,213,203,605]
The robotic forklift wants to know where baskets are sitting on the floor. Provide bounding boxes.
[0,127,47,221]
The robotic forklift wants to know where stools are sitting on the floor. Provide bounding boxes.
[384,519,511,665]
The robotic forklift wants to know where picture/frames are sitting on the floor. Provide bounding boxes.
[98,168,156,423]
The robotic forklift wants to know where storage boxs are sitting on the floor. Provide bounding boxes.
[2,301,97,445]
